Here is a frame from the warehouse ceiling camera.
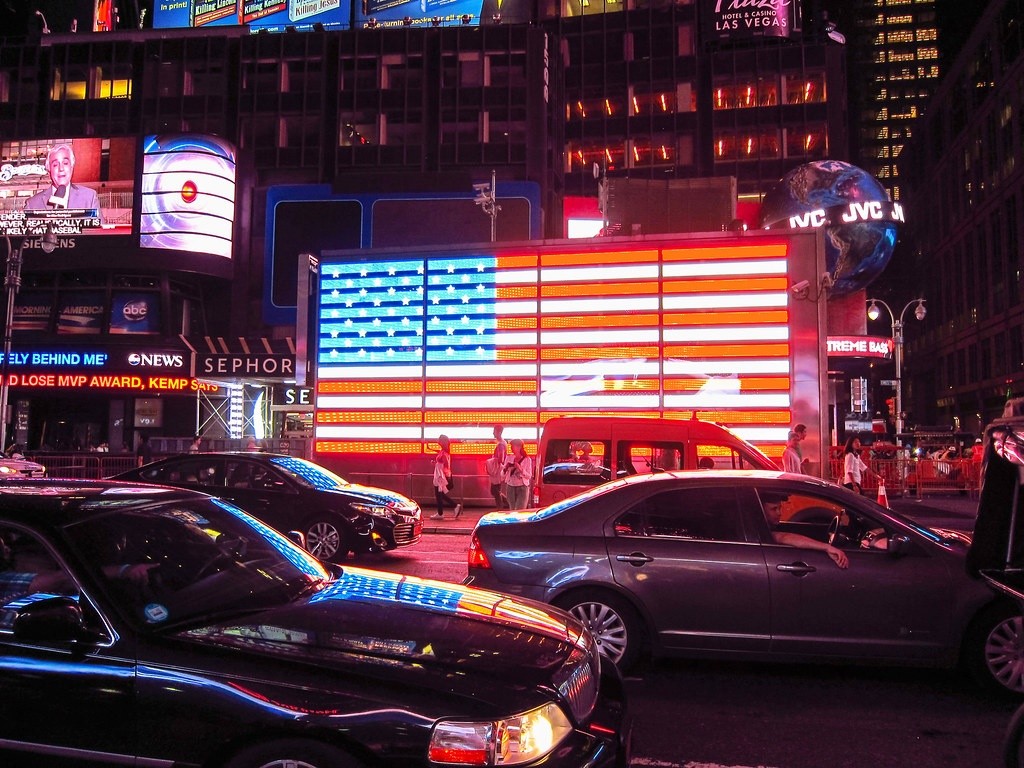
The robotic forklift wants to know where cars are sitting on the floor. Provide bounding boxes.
[461,469,1024,703]
[0,479,633,768]
[0,450,48,479]
[103,452,425,563]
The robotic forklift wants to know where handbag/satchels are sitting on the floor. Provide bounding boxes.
[447,477,453,490]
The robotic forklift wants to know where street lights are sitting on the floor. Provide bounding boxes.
[0,222,60,453]
[870,298,928,479]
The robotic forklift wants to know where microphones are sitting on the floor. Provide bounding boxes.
[48,185,66,210]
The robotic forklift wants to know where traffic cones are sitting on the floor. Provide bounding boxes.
[876,478,888,507]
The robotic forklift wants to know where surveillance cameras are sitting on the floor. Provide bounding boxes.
[474,197,491,205]
[822,271,833,288]
[473,183,490,190]
[792,280,809,293]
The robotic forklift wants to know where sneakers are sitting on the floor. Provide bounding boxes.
[454,504,462,518]
[430,513,444,520]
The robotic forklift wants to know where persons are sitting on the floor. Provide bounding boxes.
[1,431,297,634]
[425,424,531,518]
[569,425,987,568]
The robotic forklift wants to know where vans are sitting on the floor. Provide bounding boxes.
[530,417,849,526]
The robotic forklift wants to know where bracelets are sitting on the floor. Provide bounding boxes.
[118,564,131,578]
